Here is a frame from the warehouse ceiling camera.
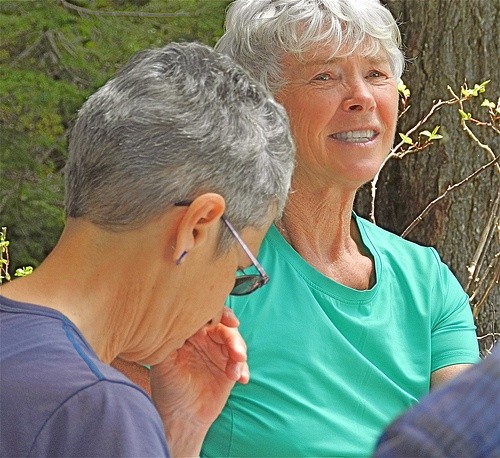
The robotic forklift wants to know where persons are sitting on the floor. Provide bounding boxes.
[373,340,500,458]
[109,1,482,458]
[0,42,298,458]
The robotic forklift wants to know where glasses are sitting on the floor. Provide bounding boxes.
[174,200,269,297]
